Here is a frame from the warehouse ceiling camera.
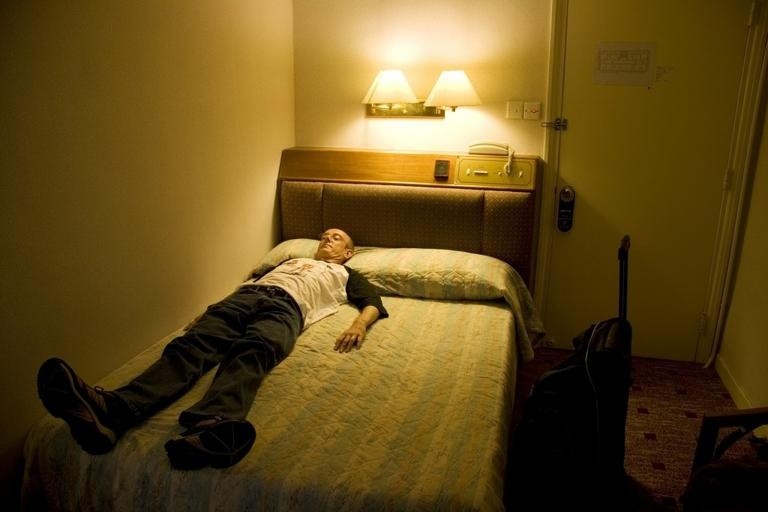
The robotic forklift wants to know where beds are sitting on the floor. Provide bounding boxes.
[21,144,541,512]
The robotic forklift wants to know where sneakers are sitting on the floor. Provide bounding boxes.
[37,357,117,455]
[164,416,256,471]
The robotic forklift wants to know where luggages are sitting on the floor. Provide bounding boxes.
[529,236,632,464]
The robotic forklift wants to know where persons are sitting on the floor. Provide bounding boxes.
[37,228,389,471]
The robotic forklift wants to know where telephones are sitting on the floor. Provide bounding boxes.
[468,142,508,155]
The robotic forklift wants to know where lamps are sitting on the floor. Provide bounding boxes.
[363,68,482,119]
